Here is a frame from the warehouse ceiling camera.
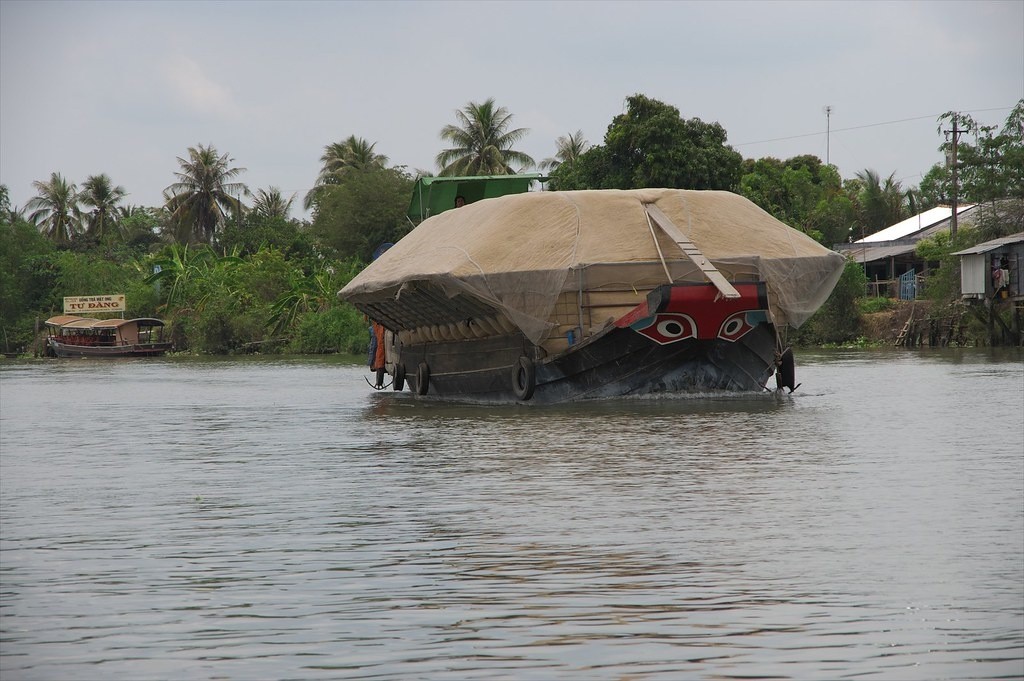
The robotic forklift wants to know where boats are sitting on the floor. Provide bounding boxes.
[41,315,174,358]
[335,172,849,403]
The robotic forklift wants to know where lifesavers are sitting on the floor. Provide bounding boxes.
[415,363,431,395]
[511,357,535,401]
[392,363,405,392]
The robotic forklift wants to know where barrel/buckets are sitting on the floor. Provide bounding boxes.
[1001,291,1008,299]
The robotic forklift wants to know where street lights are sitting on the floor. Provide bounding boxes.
[825,103,834,165]
